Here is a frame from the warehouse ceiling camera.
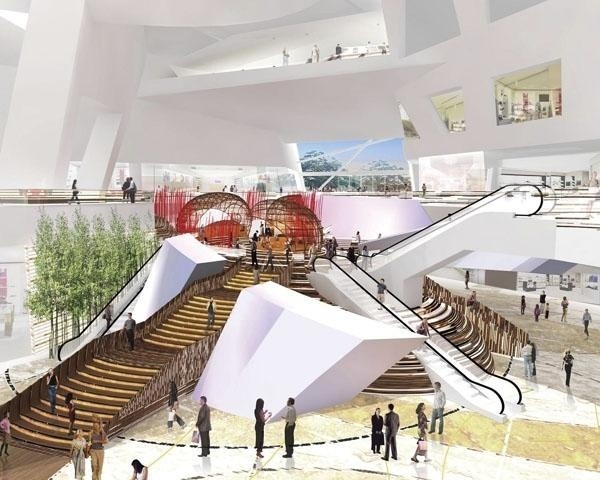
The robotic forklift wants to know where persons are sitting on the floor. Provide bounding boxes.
[70,179,80,205]
[46,368,59,414]
[520,290,592,386]
[104,305,112,329]
[497,101,504,120]
[0,411,13,457]
[124,313,136,351]
[281,398,297,458]
[281,40,388,66]
[167,380,187,430]
[88,420,108,480]
[68,429,88,480]
[205,297,216,328]
[131,459,148,480]
[416,318,431,338]
[65,392,76,436]
[195,396,212,458]
[200,223,369,274]
[222,181,427,198]
[376,278,386,311]
[370,382,445,463]
[121,176,137,204]
[465,271,470,289]
[254,398,271,458]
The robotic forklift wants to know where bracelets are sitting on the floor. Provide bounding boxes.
[96,440,99,444]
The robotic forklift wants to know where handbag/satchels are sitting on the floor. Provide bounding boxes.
[191,430,200,443]
[419,440,428,451]
[372,431,384,445]
